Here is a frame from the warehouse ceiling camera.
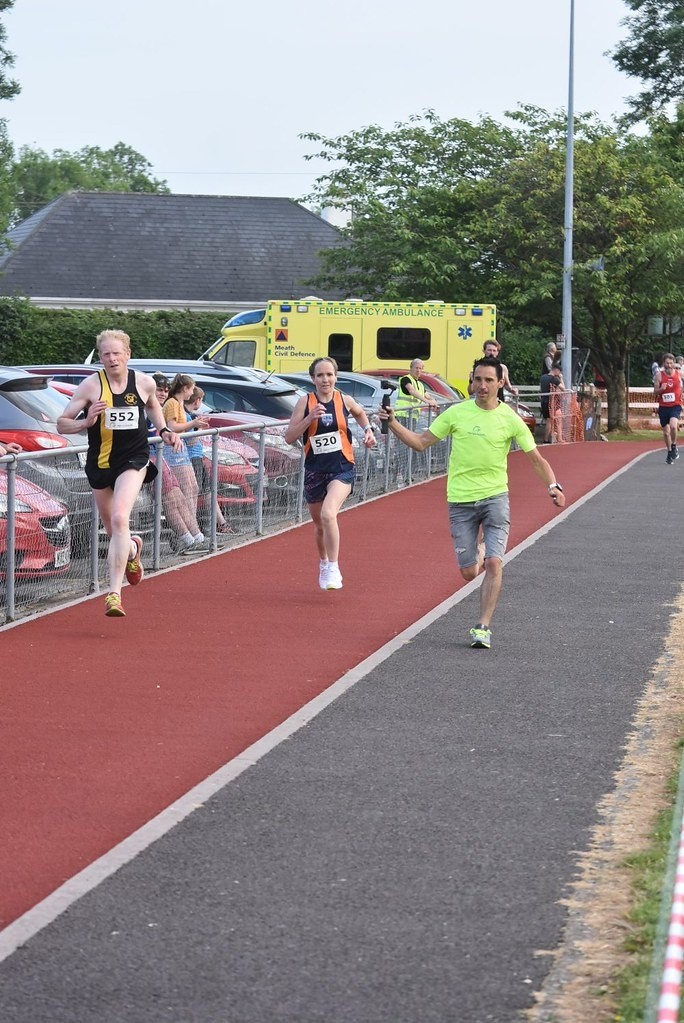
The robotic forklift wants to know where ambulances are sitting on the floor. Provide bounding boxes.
[195,296,499,399]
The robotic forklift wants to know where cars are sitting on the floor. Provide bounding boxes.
[0,466,73,580]
[15,355,472,512]
[504,394,537,434]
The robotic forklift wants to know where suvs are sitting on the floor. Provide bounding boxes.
[0,365,155,545]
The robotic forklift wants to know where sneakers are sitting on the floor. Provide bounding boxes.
[105,593,127,616]
[470,623,492,649]
[326,563,343,590]
[666,451,673,464]
[318,562,329,590]
[671,443,679,459]
[125,537,144,586]
[216,523,243,537]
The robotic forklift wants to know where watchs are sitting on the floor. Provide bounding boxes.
[547,482,563,493]
[159,427,172,438]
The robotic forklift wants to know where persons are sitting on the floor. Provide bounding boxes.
[0,442,22,457]
[467,340,684,466]
[391,358,440,487]
[56,330,185,617]
[141,371,243,555]
[378,355,567,648]
[285,357,377,592]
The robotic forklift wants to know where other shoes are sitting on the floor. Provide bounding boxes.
[543,440,551,444]
[181,537,225,553]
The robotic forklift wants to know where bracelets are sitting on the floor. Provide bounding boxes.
[363,424,374,434]
